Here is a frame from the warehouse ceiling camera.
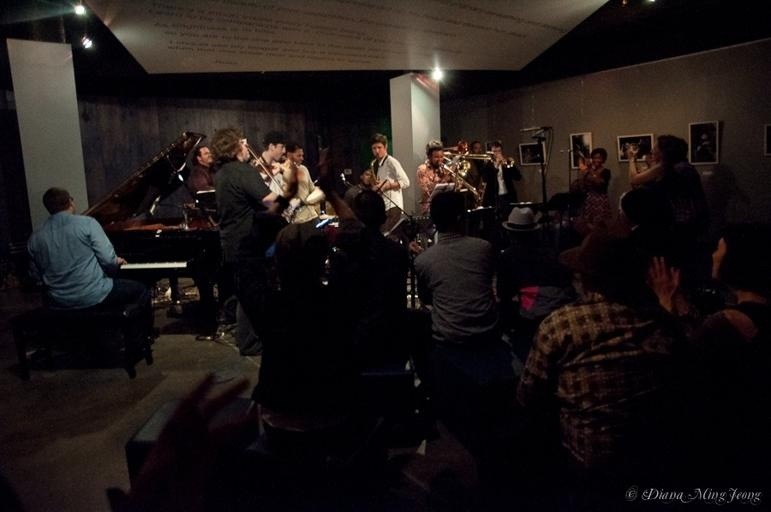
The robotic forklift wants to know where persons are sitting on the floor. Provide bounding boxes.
[24,185,152,367]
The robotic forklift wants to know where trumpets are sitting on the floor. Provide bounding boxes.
[497,157,514,168]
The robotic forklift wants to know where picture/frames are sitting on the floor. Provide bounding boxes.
[517,140,548,166]
[615,132,658,166]
[568,129,595,170]
[688,117,722,167]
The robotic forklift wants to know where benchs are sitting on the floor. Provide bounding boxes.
[4,301,158,385]
[120,315,770,510]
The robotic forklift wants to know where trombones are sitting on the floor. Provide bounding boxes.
[438,163,477,192]
[447,153,493,162]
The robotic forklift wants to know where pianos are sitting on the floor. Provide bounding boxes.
[81,131,220,338]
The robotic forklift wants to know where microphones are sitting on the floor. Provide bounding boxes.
[520,126,552,132]
[376,178,387,193]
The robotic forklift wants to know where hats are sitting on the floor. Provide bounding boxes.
[501,207,543,232]
